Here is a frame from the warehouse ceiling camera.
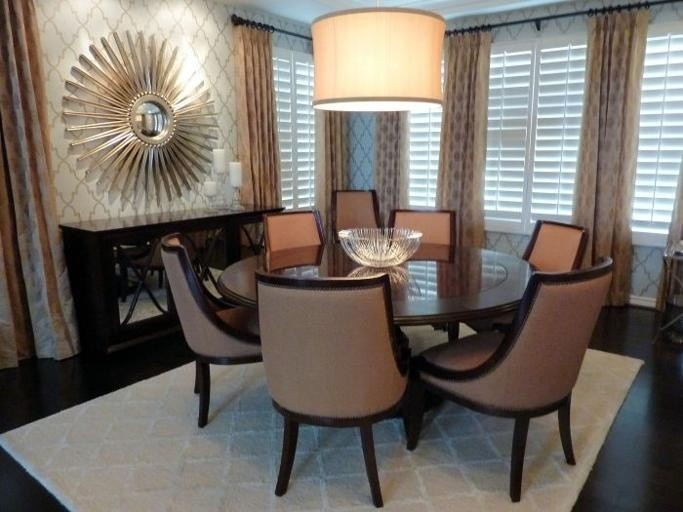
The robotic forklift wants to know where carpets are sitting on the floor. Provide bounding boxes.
[0,321,646,512]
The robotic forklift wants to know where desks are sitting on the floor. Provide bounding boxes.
[650,244,682,346]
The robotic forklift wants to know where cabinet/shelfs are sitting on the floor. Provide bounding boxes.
[56,203,285,362]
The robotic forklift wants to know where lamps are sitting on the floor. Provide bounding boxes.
[309,0,446,114]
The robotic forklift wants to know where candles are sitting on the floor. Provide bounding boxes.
[212,147,225,174]
[228,161,241,186]
[204,177,216,196]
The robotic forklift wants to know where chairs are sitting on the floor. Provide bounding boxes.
[116,225,223,328]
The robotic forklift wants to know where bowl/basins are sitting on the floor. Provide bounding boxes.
[338,226,423,268]
